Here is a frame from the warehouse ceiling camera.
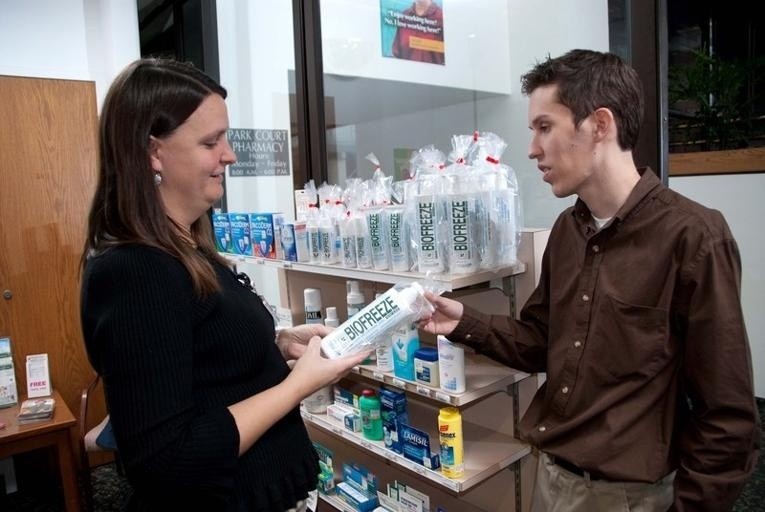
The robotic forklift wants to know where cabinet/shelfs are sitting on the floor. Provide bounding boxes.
[225,252,532,511]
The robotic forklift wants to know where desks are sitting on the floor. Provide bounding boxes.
[0,388,84,511]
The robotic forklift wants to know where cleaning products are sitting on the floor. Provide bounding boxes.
[301,286,323,325]
[344,279,372,366]
[322,305,340,329]
[436,334,468,395]
[390,323,421,382]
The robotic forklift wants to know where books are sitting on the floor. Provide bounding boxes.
[16,396,57,426]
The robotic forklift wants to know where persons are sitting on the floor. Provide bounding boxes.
[412,50,765,511]
[75,58,377,512]
[390,1,445,66]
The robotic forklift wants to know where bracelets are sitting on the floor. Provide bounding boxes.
[274,327,282,345]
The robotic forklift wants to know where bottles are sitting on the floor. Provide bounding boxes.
[305,386,334,415]
[346,280,366,319]
[305,186,516,274]
[325,307,341,329]
[437,406,465,479]
[303,288,325,326]
[358,388,384,442]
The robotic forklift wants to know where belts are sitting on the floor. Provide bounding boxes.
[554,456,600,480]
